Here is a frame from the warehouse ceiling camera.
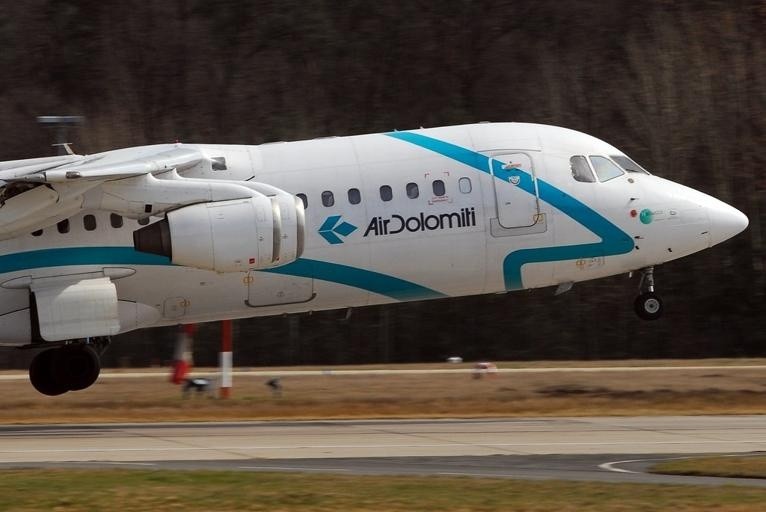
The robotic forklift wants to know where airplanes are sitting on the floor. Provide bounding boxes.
[0,122,750,397]
